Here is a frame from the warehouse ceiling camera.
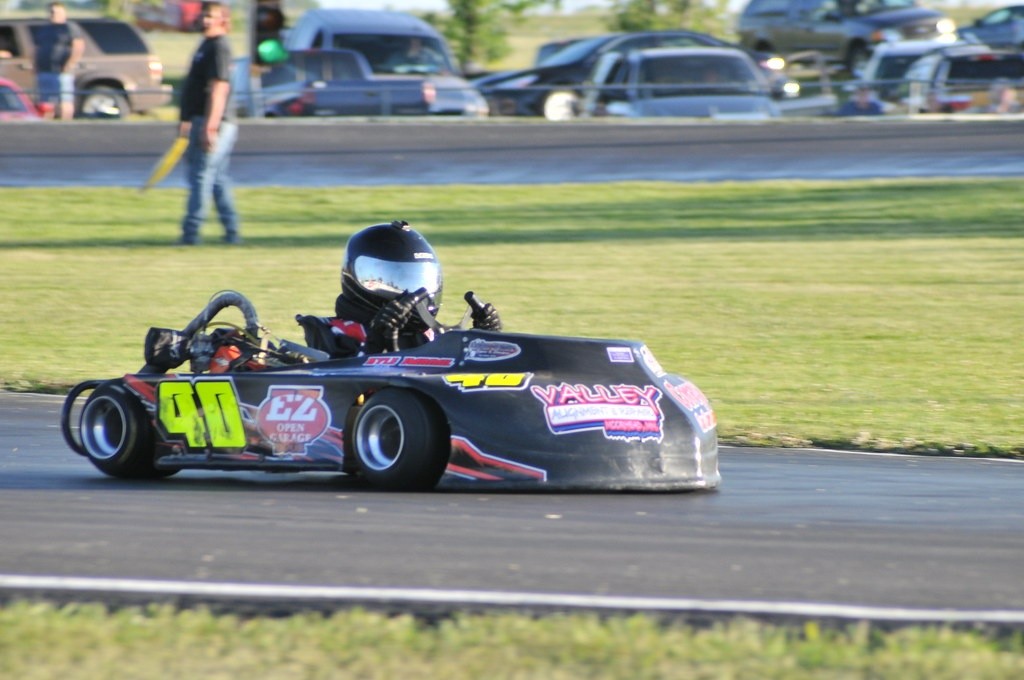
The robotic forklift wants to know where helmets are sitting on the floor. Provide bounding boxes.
[335,220,443,334]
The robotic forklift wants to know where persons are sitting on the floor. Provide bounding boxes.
[836,85,881,115]
[300,218,501,359]
[968,85,1024,114]
[33,3,86,120]
[178,0,239,245]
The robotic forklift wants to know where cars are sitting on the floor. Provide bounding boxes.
[738,0,956,79]
[1,76,56,120]
[471,6,1023,118]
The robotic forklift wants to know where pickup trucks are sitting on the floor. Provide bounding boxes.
[260,46,434,116]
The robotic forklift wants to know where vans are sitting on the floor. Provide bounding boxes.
[0,17,175,118]
[284,8,489,121]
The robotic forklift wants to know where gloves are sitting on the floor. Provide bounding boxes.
[471,303,501,331]
[365,300,412,355]
